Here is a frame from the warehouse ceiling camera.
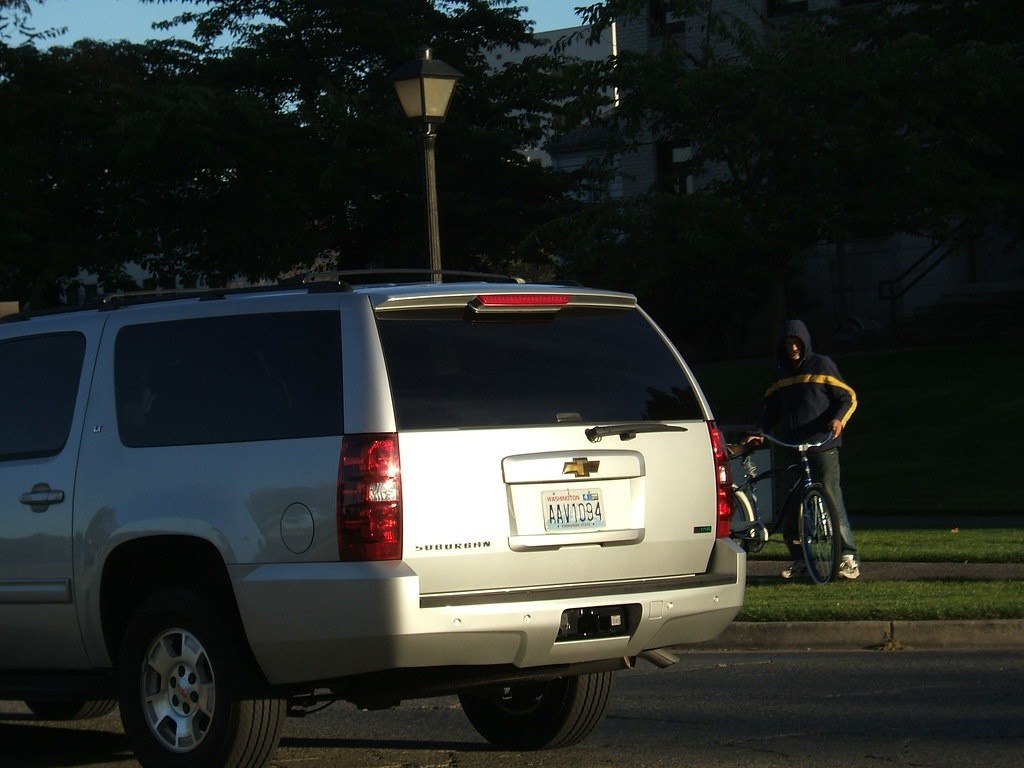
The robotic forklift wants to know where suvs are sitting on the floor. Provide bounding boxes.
[1,269,748,768]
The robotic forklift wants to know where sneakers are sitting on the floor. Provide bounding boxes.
[781,569,794,579]
[836,554,860,580]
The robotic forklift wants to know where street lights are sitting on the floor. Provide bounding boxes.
[394,46,463,282]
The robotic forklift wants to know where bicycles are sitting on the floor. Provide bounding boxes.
[729,425,843,586]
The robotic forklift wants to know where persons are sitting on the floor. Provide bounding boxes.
[739,319,859,581]
